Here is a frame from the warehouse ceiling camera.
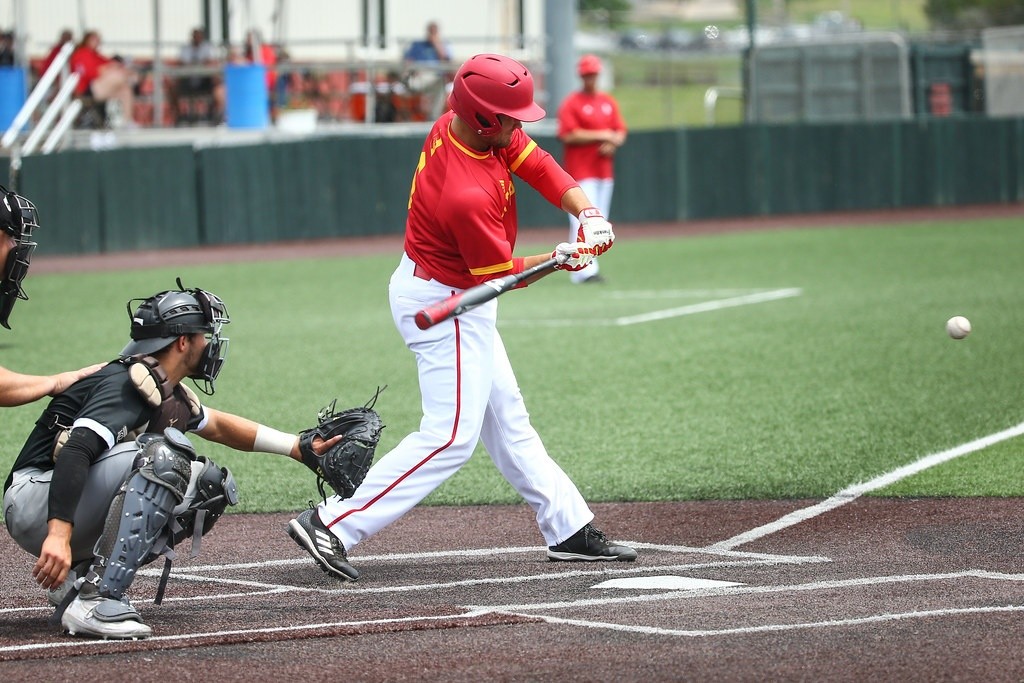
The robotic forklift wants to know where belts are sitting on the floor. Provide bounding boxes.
[414,264,432,281]
[4,473,13,496]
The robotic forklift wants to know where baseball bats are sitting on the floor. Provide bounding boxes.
[414,254,570,331]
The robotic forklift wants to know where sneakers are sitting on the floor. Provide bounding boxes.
[285,500,360,580]
[47,577,74,609]
[61,595,152,641]
[547,522,637,559]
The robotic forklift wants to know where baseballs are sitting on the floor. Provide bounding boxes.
[945,316,972,340]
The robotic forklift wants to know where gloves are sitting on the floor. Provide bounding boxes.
[551,243,594,271]
[577,207,615,256]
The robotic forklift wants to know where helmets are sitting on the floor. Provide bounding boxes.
[448,54,546,137]
[0,185,39,237]
[118,288,214,357]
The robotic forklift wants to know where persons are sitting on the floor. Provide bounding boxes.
[557,55,627,285]
[4,276,383,640]
[288,53,638,583]
[0,188,107,408]
[1,21,455,129]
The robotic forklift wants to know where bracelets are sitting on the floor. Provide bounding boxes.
[252,424,297,455]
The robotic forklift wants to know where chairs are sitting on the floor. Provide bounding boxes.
[25,57,420,131]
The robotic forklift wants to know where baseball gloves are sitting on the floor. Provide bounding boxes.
[298,406,383,501]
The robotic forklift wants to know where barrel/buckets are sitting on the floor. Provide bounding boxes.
[0,68,25,138]
[223,65,266,132]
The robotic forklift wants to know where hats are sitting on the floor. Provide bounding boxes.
[578,56,601,74]
